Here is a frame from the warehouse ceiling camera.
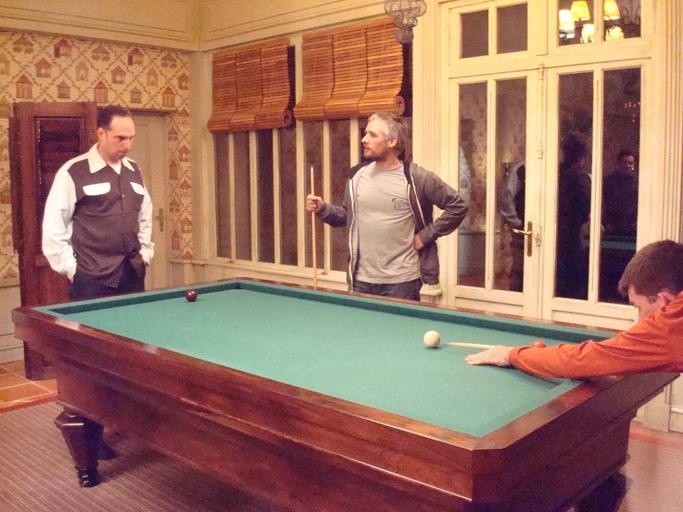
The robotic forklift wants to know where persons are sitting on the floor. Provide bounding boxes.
[501,129,639,297]
[305,111,468,302]
[466,238,683,379]
[41,102,154,460]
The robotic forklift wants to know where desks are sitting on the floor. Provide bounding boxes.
[11,273,683,510]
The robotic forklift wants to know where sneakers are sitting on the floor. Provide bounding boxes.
[95,435,114,460]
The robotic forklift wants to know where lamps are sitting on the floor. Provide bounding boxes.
[557,0,626,48]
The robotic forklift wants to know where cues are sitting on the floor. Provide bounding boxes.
[445,343,496,349]
[311,166,317,291]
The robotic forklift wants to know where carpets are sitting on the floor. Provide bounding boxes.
[0,359,683,512]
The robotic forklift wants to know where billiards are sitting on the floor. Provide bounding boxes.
[424,330,440,348]
[185,290,197,302]
